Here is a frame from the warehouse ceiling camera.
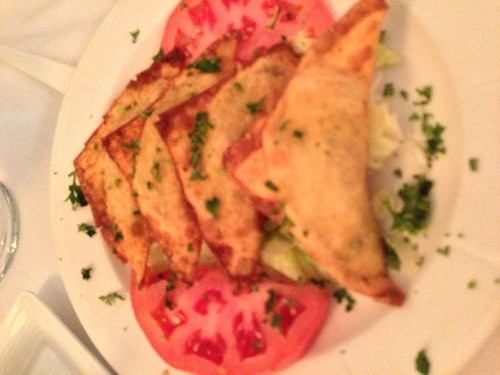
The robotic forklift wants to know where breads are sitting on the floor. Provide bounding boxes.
[71,1,408,308]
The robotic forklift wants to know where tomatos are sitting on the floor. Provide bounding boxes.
[159,1,338,63]
[130,266,330,375]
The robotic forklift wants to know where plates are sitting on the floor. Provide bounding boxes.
[0,291,113,375]
[50,0,500,375]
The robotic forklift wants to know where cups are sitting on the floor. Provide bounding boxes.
[0,181,19,282]
